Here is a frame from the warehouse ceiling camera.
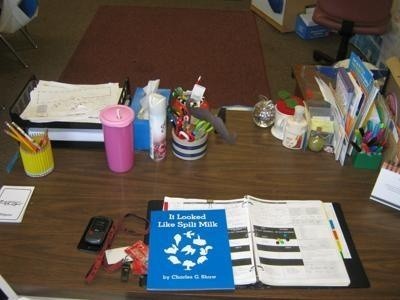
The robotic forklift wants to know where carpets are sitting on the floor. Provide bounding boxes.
[57,5,270,109]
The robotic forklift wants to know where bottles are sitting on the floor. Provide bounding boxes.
[149,93,167,162]
[271,96,308,149]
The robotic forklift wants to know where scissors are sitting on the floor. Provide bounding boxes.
[195,120,213,141]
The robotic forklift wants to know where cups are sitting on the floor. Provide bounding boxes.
[19,136,54,178]
[172,126,208,161]
[99,105,136,174]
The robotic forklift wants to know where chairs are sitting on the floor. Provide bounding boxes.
[312,0,392,64]
[0,0,41,68]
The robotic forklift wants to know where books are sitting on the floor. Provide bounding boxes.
[314,53,381,167]
[140,194,370,292]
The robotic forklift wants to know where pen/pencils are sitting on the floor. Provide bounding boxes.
[167,75,209,142]
[350,121,387,155]
[7,151,20,174]
[3,121,48,153]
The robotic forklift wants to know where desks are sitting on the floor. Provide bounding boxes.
[0,111,400,299]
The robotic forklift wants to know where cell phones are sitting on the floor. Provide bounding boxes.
[77,216,113,253]
